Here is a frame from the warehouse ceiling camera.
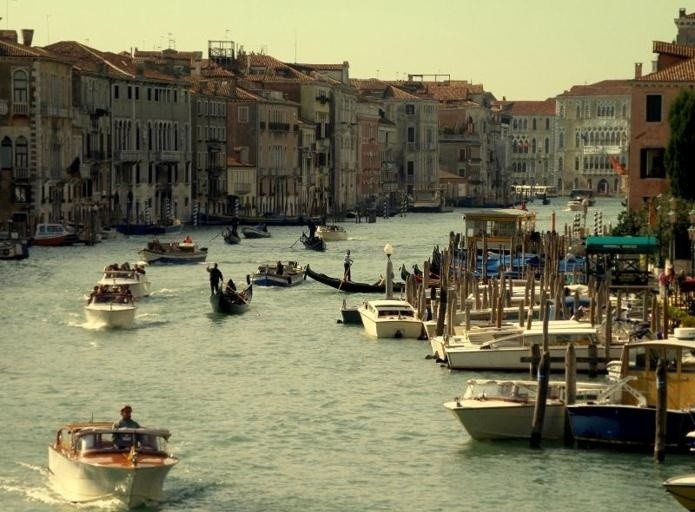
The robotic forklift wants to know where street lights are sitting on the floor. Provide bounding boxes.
[656,204,663,267]
[669,210,677,266]
[686,209,695,278]
[384,243,393,302]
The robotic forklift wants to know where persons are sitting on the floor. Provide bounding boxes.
[343,250,353,281]
[88,261,146,305]
[226,278,236,293]
[231,214,239,234]
[183,235,192,243]
[111,406,154,450]
[276,261,283,275]
[261,222,267,232]
[206,263,223,295]
[307,219,316,237]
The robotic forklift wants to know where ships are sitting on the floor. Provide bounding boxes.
[516,184,559,197]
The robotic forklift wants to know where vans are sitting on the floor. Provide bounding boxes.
[36,224,74,238]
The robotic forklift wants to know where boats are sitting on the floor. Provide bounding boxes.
[444,320,645,370]
[444,378,621,439]
[210,284,253,314]
[464,208,536,250]
[139,248,208,265]
[85,293,136,326]
[254,264,303,286]
[101,220,185,239]
[99,270,152,299]
[304,237,326,251]
[566,328,695,446]
[306,264,406,293]
[317,224,348,241]
[225,232,242,245]
[1,241,29,260]
[567,199,582,211]
[242,226,271,238]
[571,189,596,205]
[542,198,550,204]
[358,300,423,339]
[49,422,178,510]
